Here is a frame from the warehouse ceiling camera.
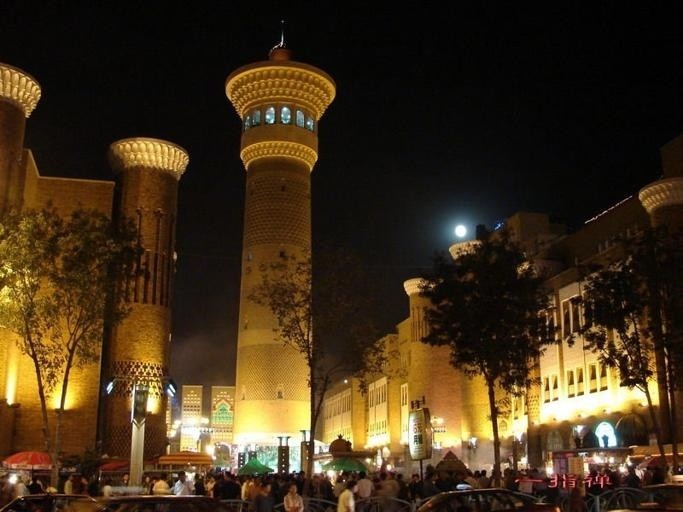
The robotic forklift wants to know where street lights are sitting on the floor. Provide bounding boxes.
[106,374,177,487]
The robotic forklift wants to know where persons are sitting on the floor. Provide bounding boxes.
[1,462,683,511]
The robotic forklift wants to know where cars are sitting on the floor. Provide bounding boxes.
[0,484,683,512]
